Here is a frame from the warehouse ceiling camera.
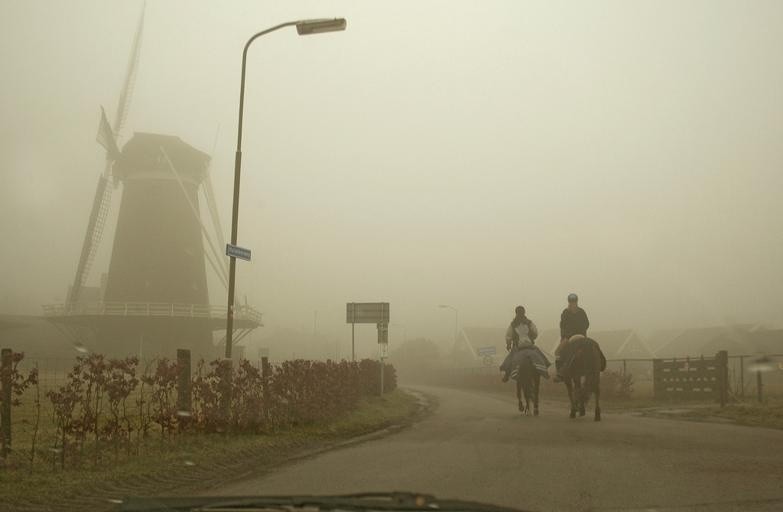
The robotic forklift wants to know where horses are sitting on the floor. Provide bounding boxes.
[564,337,603,422]
[514,348,541,416]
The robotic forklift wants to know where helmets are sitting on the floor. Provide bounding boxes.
[568,294,577,301]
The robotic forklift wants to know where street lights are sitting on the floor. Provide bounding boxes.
[438,303,457,351]
[223,14,359,426]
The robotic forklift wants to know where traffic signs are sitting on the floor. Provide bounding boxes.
[223,243,251,261]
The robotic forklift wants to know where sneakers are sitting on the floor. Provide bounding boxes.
[501,374,510,381]
[554,374,563,381]
[544,371,550,379]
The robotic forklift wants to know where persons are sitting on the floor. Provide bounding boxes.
[501,306,552,383]
[552,293,590,385]
